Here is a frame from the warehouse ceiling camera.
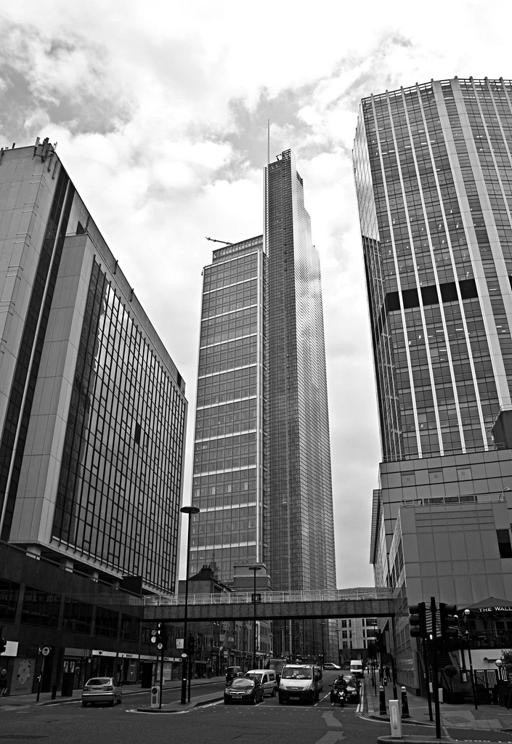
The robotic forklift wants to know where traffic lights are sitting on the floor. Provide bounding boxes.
[0,624,8,654]
[439,601,460,639]
[148,621,169,653]
[176,639,183,649]
[187,637,195,655]
[408,601,428,640]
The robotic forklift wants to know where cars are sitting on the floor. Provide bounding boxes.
[223,658,366,707]
[78,675,122,707]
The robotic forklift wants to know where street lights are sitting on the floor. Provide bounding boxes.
[465,630,479,711]
[177,504,201,706]
[248,566,262,669]
[495,658,504,681]
[455,608,471,670]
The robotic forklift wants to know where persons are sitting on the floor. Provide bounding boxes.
[329,673,350,708]
[378,665,386,682]
[385,665,392,682]
[0,668,9,698]
